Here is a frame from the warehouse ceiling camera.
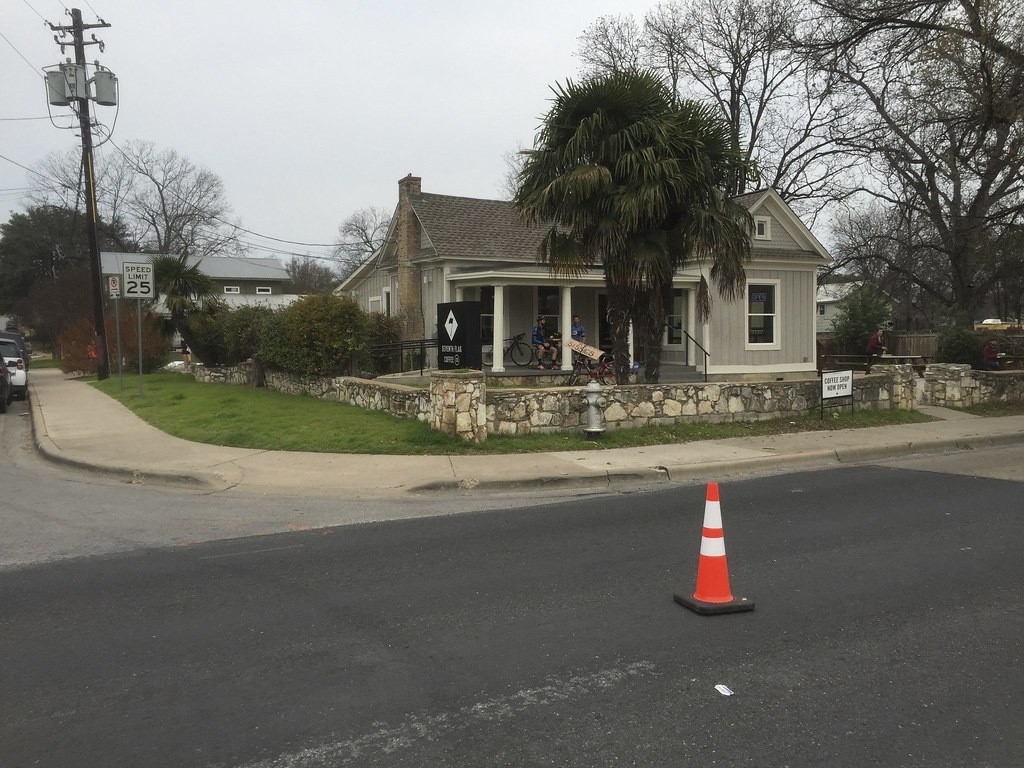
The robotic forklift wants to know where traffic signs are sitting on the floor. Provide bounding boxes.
[109,276,121,299]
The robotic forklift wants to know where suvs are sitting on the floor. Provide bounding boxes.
[0,338,27,401]
[0,330,33,373]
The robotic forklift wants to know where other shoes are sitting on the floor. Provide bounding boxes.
[537,365,545,370]
[551,365,560,370]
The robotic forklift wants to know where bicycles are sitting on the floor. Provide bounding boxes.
[534,332,563,369]
[568,350,617,386]
[481,331,534,367]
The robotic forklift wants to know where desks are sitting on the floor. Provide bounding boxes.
[547,338,562,342]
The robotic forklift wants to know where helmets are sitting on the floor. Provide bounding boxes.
[536,315,545,321]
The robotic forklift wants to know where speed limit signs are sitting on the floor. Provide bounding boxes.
[121,261,155,299]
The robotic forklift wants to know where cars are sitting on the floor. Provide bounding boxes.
[0,354,17,414]
[5,320,18,331]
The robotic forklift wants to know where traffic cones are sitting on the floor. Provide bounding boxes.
[673,479,756,619]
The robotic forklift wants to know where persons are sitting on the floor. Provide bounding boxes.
[531,315,561,370]
[864,327,887,375]
[571,315,596,369]
[983,339,1002,371]
[181,337,192,366]
